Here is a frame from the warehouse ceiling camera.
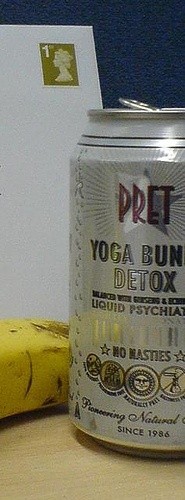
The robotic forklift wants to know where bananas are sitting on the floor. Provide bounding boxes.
[1,319,69,423]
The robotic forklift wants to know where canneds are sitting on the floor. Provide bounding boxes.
[68,98,185,460]
